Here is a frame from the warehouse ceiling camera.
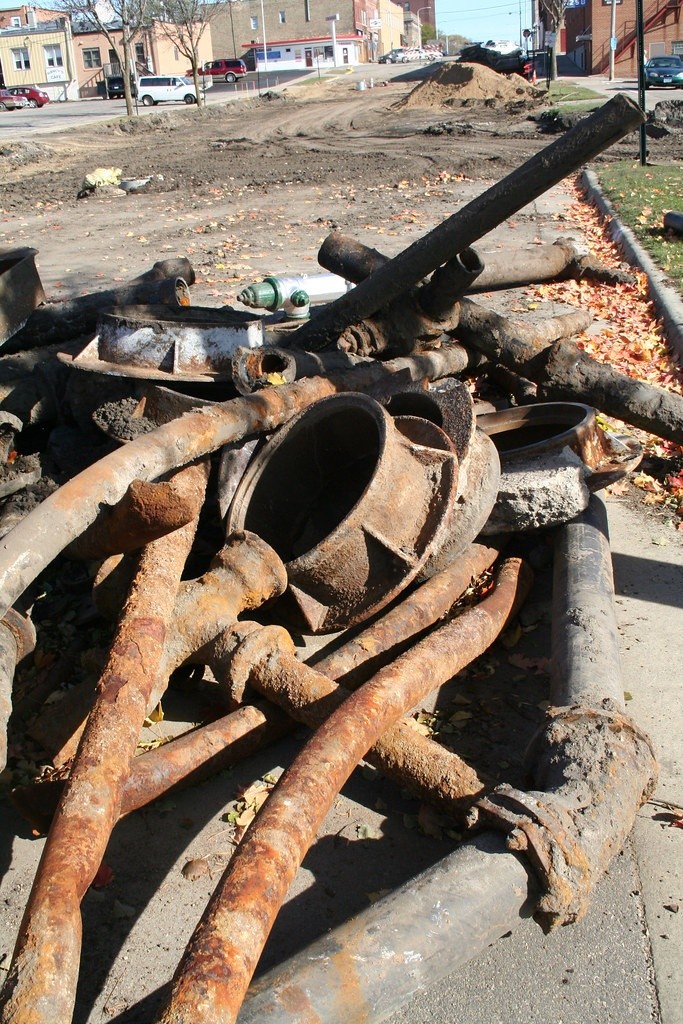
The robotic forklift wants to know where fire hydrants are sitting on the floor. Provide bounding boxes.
[236,272,356,322]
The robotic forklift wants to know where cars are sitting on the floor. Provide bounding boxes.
[642,54,683,90]
[0,89,29,111]
[377,48,443,64]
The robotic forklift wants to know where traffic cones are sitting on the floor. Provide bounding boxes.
[532,69,536,82]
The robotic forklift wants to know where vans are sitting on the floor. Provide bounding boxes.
[136,75,205,106]
[6,87,48,108]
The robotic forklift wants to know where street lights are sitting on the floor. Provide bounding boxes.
[509,12,522,47]
[417,6,431,48]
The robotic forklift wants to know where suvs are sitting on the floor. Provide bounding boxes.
[108,76,135,100]
[184,59,247,83]
